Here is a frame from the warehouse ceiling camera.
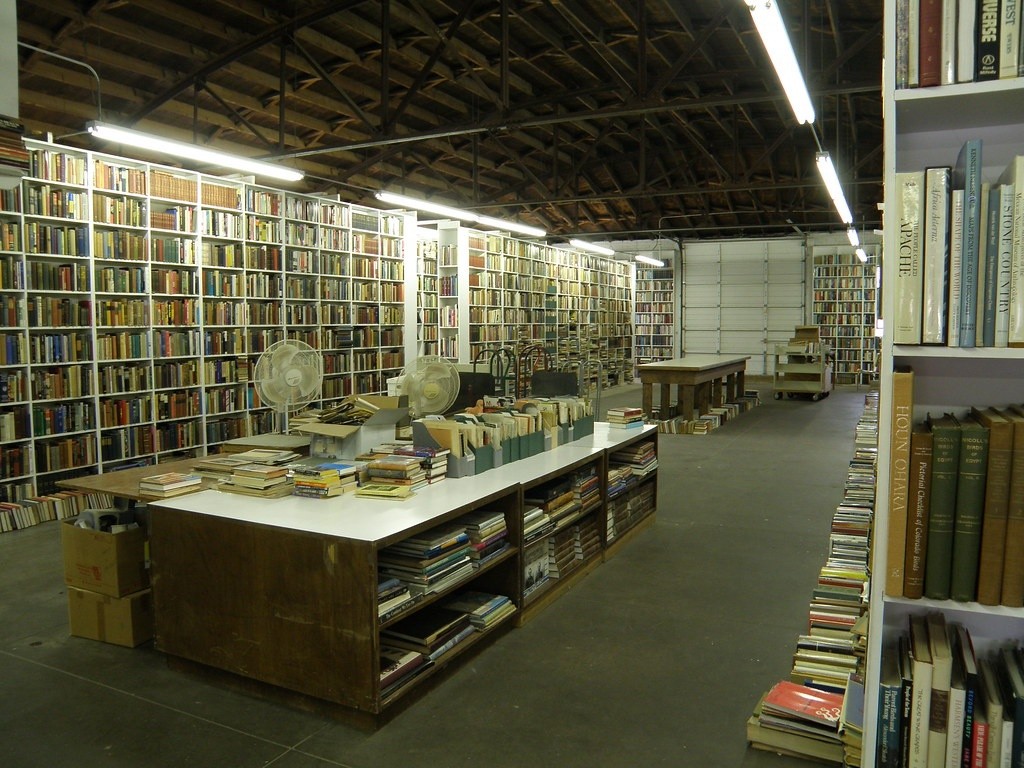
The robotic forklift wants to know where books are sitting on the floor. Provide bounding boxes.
[807,253,880,384]
[896,0,1024,90]
[886,362,1024,611]
[746,385,879,768]
[876,612,1024,768]
[0,109,763,705]
[891,138,1024,347]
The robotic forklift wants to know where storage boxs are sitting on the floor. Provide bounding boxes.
[67,585,151,647]
[292,394,412,461]
[387,374,407,396]
[62,519,150,598]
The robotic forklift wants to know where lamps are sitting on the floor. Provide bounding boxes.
[374,0,547,239]
[85,0,306,185]
[635,223,664,266]
[743,0,867,262]
[569,120,615,256]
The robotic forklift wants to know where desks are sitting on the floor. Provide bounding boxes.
[55,453,238,502]
[634,355,751,422]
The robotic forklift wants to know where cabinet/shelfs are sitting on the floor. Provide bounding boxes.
[811,244,880,387]
[0,136,682,499]
[147,480,521,715]
[560,421,658,551]
[860,0,1024,768]
[447,446,606,627]
[773,341,825,400]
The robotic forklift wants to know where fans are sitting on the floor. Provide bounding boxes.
[253,339,323,434]
[395,355,460,426]
